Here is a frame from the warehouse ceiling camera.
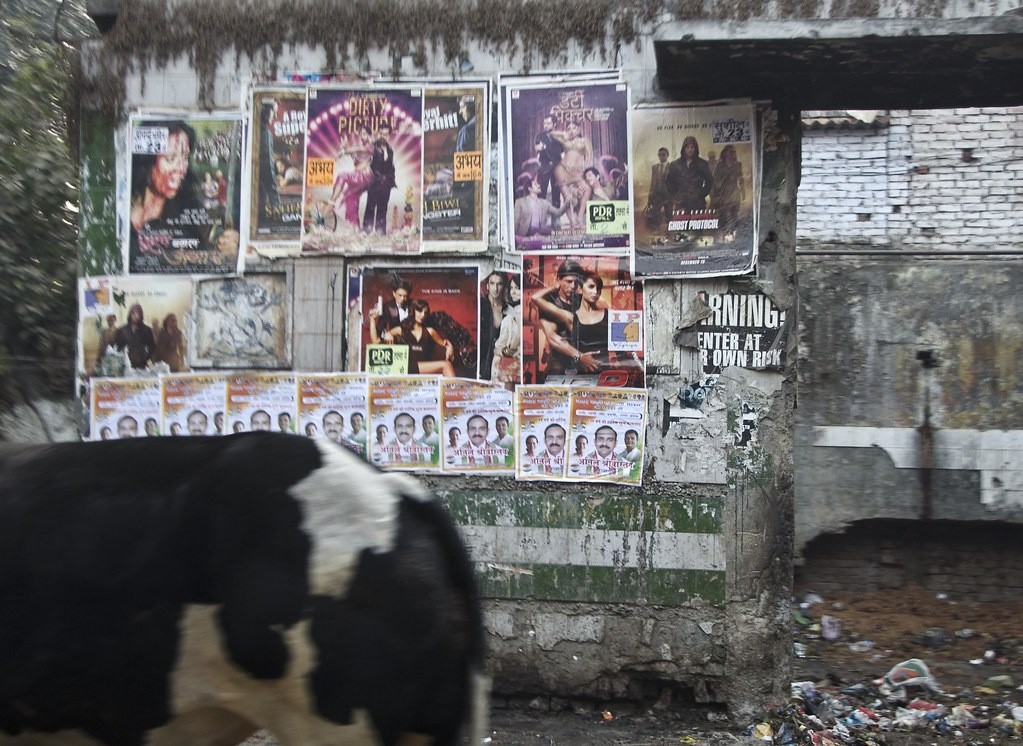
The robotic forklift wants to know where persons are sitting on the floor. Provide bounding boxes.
[93,304,187,374]
[369,277,456,377]
[647,136,745,227]
[234,410,640,477]
[130,120,243,262]
[327,121,397,236]
[97,409,161,440]
[454,95,475,226]
[531,259,628,375]
[514,113,622,237]
[169,411,224,437]
[480,270,521,391]
[259,95,303,226]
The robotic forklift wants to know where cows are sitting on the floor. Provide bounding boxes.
[0,428,490,745]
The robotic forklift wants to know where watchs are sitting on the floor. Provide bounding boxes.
[573,351,581,364]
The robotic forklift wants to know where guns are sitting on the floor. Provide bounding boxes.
[369,295,384,317]
